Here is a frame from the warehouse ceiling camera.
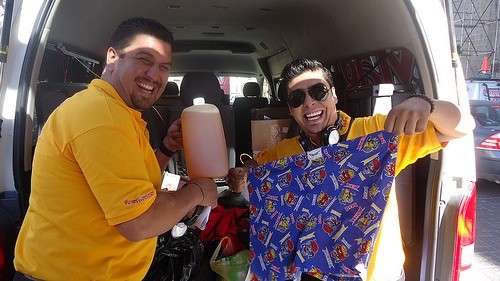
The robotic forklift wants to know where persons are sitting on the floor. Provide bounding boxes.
[13,17,217,281]
[227,57,475,281]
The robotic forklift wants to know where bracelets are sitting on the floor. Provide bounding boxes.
[159,142,174,157]
[186,182,205,205]
[408,94,435,114]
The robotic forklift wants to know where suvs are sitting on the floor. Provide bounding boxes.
[464,55,500,184]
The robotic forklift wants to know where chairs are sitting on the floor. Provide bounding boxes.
[232,82,268,141]
[169,72,235,144]
[143,82,179,147]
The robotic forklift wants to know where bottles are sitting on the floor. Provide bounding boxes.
[181,96,229,180]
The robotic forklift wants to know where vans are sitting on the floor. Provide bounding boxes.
[0,0,476,281]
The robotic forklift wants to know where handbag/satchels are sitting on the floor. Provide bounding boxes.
[209,236,250,281]
[145,227,206,281]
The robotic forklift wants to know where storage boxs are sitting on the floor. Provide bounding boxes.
[250,107,291,157]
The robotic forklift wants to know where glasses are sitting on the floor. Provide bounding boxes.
[287,82,333,108]
